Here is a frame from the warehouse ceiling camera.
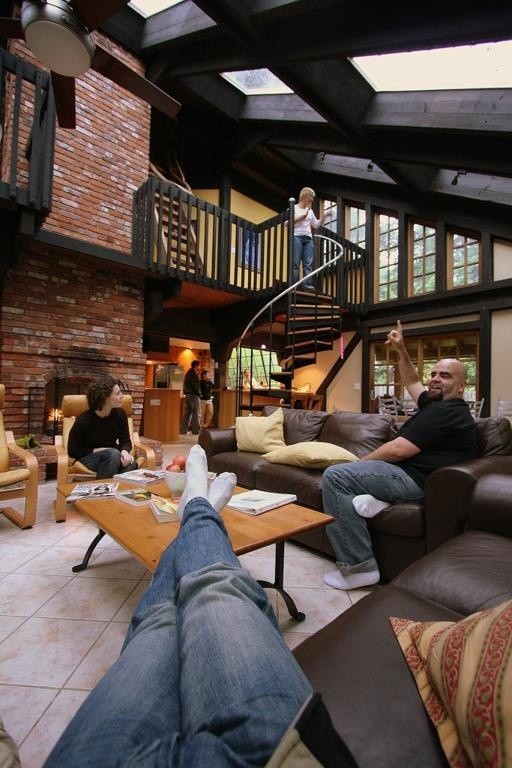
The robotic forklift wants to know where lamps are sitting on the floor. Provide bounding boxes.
[20,4,96,79]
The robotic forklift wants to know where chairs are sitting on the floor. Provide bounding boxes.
[54,395,155,522]
[1,385,39,529]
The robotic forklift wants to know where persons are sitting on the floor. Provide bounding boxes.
[243,369,256,390]
[67,375,138,480]
[181,360,202,435]
[41,445,354,768]
[200,370,213,431]
[284,187,325,291]
[321,320,486,591]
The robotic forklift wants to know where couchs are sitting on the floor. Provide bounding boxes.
[293,474,511,767]
[198,401,512,581]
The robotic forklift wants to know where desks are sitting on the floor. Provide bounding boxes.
[142,387,181,441]
[181,388,238,431]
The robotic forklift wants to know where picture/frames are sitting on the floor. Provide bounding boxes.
[237,220,264,275]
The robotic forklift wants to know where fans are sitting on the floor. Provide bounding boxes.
[0,0,182,129]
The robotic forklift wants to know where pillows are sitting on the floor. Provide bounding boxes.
[261,442,358,469]
[389,415,510,458]
[260,406,328,445]
[234,406,285,453]
[388,594,512,768]
[317,410,394,461]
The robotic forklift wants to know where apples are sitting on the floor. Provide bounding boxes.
[173,456,185,469]
[166,464,180,472]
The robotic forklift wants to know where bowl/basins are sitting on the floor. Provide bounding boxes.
[165,468,187,500]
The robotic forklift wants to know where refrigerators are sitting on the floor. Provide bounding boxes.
[153,366,182,392]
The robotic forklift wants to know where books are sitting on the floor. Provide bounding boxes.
[65,481,120,504]
[113,468,166,486]
[114,488,180,524]
[225,488,297,515]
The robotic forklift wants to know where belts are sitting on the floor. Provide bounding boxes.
[300,234,312,240]
